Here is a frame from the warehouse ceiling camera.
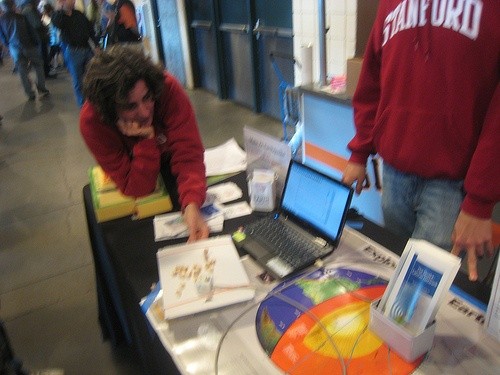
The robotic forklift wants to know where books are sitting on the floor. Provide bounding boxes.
[87,138,256,321]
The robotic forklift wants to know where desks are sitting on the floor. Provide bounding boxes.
[83,171,491,375]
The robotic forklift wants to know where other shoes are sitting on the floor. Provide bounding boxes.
[31,96,35,103]
[39,89,49,96]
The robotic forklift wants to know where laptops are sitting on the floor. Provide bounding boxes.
[232,159,354,281]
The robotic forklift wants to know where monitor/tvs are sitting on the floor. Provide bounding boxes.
[298,86,385,231]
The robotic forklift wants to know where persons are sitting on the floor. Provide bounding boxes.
[0,0,139,103]
[339,0,500,297]
[79,42,211,245]
